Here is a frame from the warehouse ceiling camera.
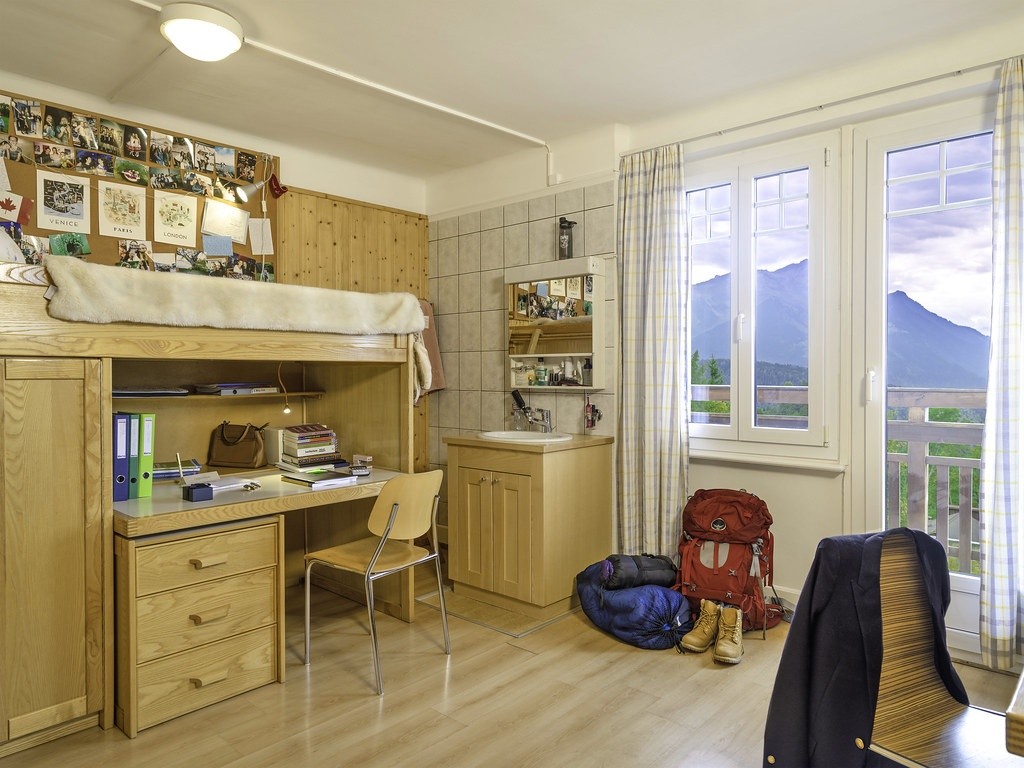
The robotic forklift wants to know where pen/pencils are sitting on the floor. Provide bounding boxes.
[176,453,183,478]
[306,470,328,474]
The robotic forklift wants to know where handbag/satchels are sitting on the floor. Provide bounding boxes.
[205,419,268,470]
[601,552,679,590]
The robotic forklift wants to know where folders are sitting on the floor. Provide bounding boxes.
[112,411,156,501]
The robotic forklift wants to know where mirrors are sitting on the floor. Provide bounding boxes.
[500,257,604,353]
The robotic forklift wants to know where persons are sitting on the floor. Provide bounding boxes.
[1,225,21,240]
[0,106,255,197]
[517,294,593,320]
[68,238,82,255]
[123,241,150,270]
[210,259,269,282]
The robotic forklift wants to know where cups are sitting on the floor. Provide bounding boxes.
[514,411,526,430]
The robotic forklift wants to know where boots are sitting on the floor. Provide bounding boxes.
[713,605,745,664]
[679,599,724,653]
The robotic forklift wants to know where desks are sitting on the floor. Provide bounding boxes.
[110,453,417,626]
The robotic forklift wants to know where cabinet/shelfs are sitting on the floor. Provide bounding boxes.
[115,512,285,742]
[0,357,105,759]
[508,353,606,389]
[444,433,616,620]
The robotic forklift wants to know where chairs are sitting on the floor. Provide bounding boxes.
[299,466,451,696]
[806,526,1024,768]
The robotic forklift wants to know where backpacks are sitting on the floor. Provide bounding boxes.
[670,488,789,641]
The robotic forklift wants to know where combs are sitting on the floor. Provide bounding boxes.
[510,389,534,424]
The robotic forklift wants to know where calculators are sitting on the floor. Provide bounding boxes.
[349,466,370,475]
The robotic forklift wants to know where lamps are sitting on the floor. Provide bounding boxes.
[153,3,244,64]
[236,151,278,203]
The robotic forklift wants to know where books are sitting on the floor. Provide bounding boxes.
[194,382,272,392]
[275,424,358,492]
[153,459,202,478]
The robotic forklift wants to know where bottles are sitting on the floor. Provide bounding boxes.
[512,404,521,413]
[559,357,592,386]
[510,412,515,430]
[526,407,543,431]
[515,358,548,386]
[559,217,577,260]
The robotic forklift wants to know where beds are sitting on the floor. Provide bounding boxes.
[0,226,419,362]
[509,314,592,333]
